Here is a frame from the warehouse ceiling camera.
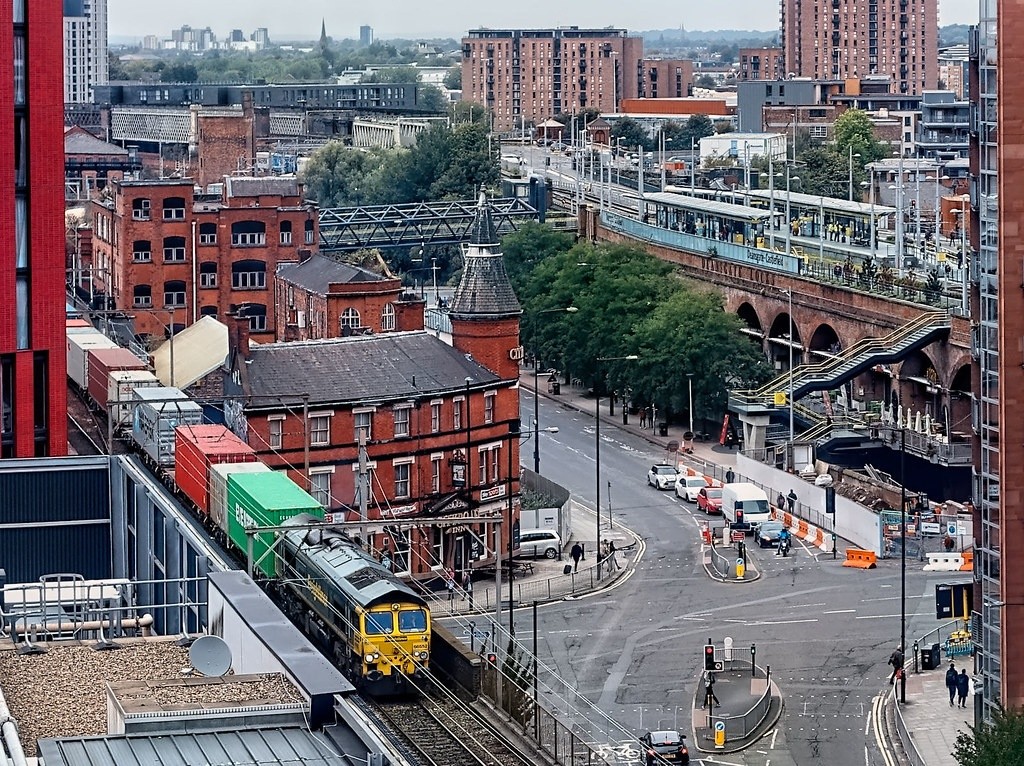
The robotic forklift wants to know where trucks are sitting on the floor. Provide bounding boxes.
[723,482,772,534]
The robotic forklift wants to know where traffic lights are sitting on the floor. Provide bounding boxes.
[826,487,835,512]
[487,652,497,670]
[730,509,750,530]
[704,645,715,672]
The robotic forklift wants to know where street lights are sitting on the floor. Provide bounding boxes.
[853,424,910,703]
[534,306,578,473]
[465,377,473,612]
[508,426,560,656]
[595,355,639,581]
[779,285,794,443]
[521,111,969,307]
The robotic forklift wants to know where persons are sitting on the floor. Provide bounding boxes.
[462,572,474,602]
[444,566,457,602]
[640,405,657,431]
[788,489,799,516]
[702,670,722,711]
[570,542,584,575]
[600,537,624,574]
[889,645,906,687]
[956,669,971,709]
[946,664,960,708]
[640,194,965,268]
[944,532,955,552]
[776,527,792,557]
[777,491,787,512]
[726,467,736,485]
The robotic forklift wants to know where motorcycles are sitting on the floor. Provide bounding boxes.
[778,535,791,556]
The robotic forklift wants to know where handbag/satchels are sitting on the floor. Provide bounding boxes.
[896,667,902,679]
[445,579,454,590]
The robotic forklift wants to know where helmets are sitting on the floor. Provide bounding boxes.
[782,527,786,532]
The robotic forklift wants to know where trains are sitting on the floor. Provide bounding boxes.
[65,301,431,697]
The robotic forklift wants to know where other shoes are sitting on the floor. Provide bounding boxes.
[889,681,894,685]
[949,700,967,709]
[714,703,721,708]
[702,705,705,710]
[602,564,622,572]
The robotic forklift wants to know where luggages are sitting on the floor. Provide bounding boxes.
[564,558,572,574]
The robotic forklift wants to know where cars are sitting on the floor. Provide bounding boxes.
[647,464,680,490]
[754,521,791,547]
[697,486,722,514]
[675,476,710,502]
[640,730,690,766]
[519,529,563,559]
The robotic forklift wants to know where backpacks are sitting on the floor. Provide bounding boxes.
[950,539,954,548]
[711,673,715,684]
